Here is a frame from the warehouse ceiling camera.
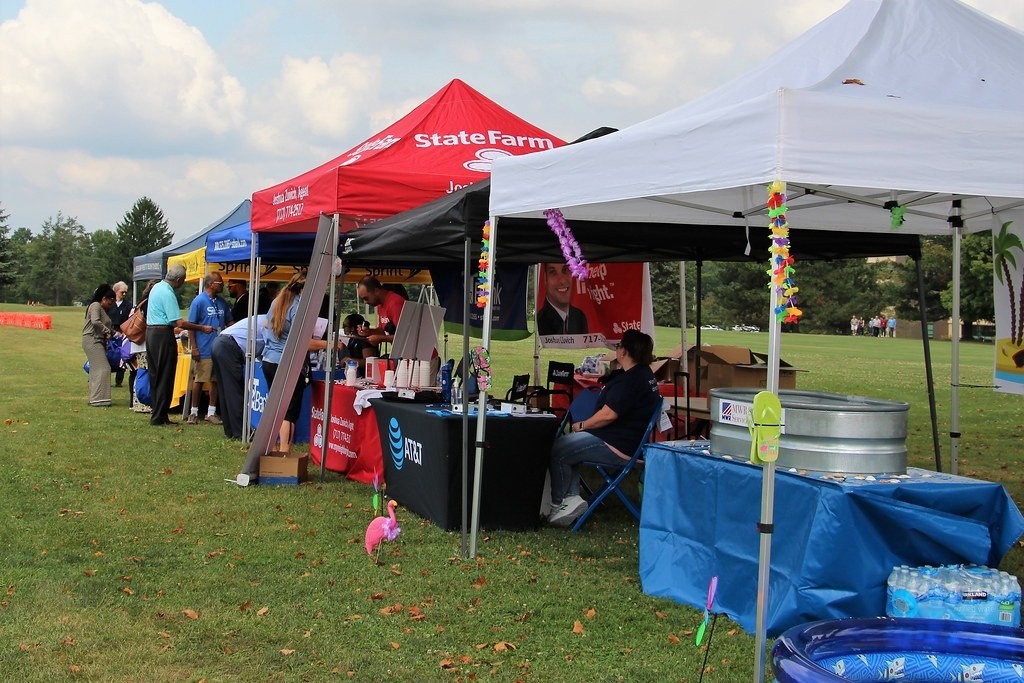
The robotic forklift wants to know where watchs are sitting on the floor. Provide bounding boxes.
[579,421,585,431]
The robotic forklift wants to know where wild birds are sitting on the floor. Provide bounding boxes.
[365,499,401,567]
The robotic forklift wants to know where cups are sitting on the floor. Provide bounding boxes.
[396,359,431,388]
[383,371,394,386]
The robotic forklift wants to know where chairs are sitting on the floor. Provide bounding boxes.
[528,361,575,421]
[571,396,664,532]
[439,356,481,401]
[490,373,530,405]
[556,386,604,443]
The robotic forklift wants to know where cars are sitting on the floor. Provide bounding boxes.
[731,325,760,332]
[701,325,724,330]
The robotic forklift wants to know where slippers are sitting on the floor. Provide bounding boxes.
[471,346,492,392]
[746,391,782,465]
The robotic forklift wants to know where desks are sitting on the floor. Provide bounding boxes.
[639,438,1024,639]
[243,360,557,530]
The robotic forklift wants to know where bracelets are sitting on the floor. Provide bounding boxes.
[383,336,386,342]
[192,348,199,350]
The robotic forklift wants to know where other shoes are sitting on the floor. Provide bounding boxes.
[150,421,178,426]
[115,384,123,387]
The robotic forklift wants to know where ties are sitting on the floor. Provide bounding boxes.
[565,316,569,334]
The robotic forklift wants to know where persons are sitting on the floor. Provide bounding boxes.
[187,270,439,454]
[547,327,661,529]
[850,315,896,338]
[537,263,589,336]
[81,263,214,426]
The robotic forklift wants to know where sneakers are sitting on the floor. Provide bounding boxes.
[204,412,223,425]
[187,414,200,424]
[547,496,588,526]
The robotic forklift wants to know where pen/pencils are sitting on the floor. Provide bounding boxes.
[425,404,450,407]
[683,445,709,447]
[361,321,365,332]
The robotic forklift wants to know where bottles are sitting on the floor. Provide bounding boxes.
[441,362,452,403]
[886,562,1022,627]
[452,378,463,403]
[346,360,356,387]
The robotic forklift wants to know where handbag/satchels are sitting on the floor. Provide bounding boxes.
[120,298,148,345]
[118,340,135,363]
[105,340,121,372]
[83,360,89,374]
[296,357,313,391]
[135,368,152,406]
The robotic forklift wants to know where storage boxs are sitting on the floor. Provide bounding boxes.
[259,451,309,485]
[599,342,808,396]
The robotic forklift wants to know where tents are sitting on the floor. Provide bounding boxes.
[242,78,561,474]
[319,122,946,556]
[468,0,1024,683]
[131,197,439,444]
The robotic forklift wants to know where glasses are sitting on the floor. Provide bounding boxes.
[119,290,128,294]
[214,282,224,286]
[228,284,238,289]
[616,343,621,349]
[360,296,369,301]
[343,322,350,329]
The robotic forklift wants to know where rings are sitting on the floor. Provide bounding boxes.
[207,330,208,331]
[572,426,573,427]
